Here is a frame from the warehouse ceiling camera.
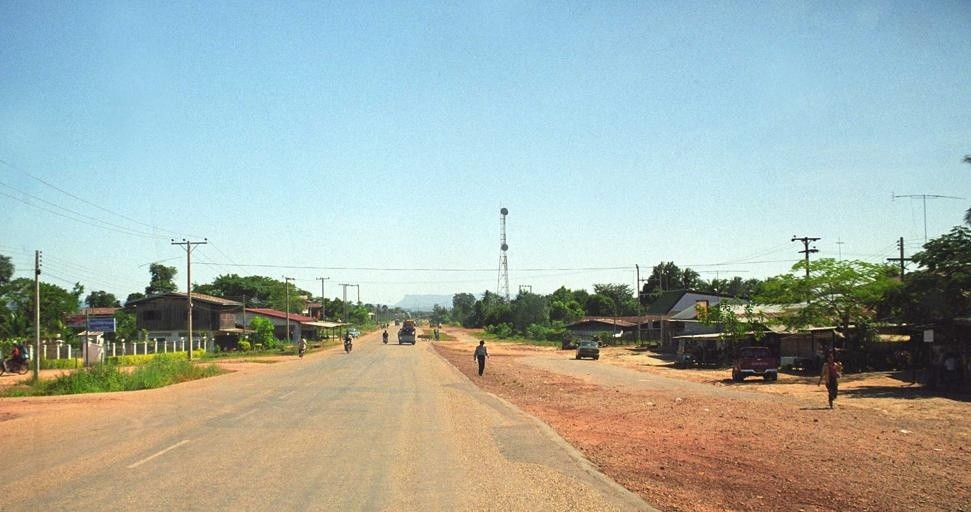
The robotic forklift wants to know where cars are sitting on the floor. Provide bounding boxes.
[575,340,600,360]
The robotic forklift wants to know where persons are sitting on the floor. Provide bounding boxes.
[298,335,307,357]
[383,330,388,343]
[342,331,353,352]
[2,340,29,374]
[474,341,490,377]
[817,355,842,409]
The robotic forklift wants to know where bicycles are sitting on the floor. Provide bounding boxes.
[298,341,307,359]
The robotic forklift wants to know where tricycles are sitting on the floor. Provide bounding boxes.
[731,345,779,383]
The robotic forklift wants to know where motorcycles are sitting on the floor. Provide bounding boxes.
[382,334,389,344]
[0,353,31,377]
[343,337,353,354]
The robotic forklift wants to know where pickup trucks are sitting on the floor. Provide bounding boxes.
[397,328,416,346]
